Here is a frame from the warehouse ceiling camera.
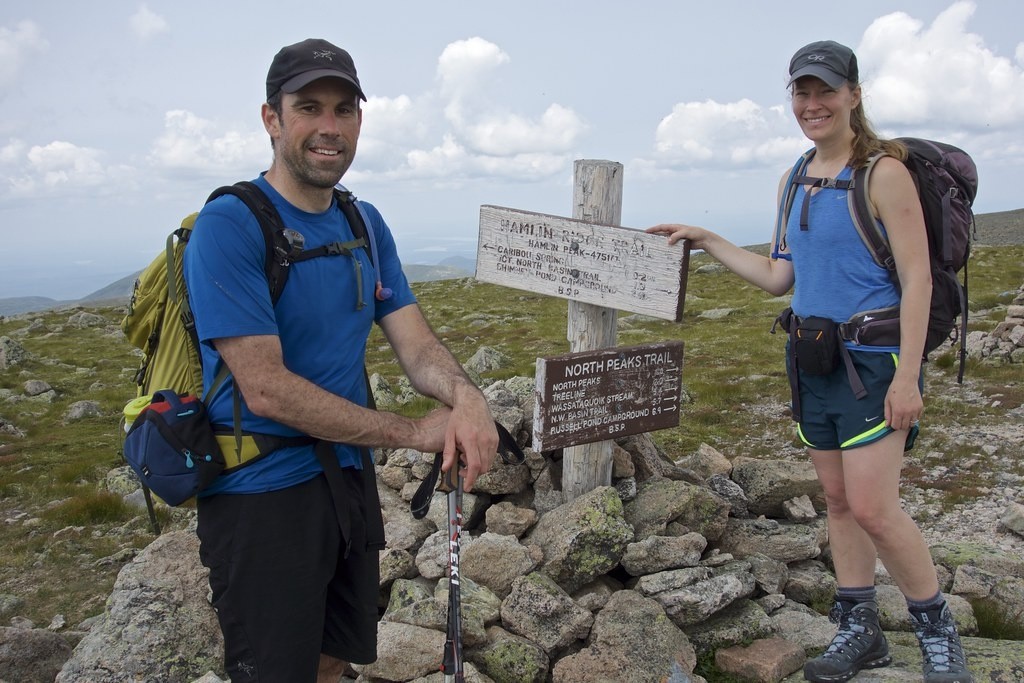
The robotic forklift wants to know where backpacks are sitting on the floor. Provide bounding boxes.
[121,180,374,507]
[781,137,978,358]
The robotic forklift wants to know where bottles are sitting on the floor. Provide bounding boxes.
[123,388,152,433]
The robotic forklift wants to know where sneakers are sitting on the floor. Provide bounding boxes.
[803,592,891,682]
[909,605,970,683]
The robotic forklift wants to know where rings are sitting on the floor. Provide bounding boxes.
[910,419,918,424]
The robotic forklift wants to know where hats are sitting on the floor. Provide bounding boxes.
[785,40,859,90]
[266,40,367,103]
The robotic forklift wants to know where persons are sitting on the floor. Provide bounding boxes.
[647,41,966,683]
[185,39,500,683]
